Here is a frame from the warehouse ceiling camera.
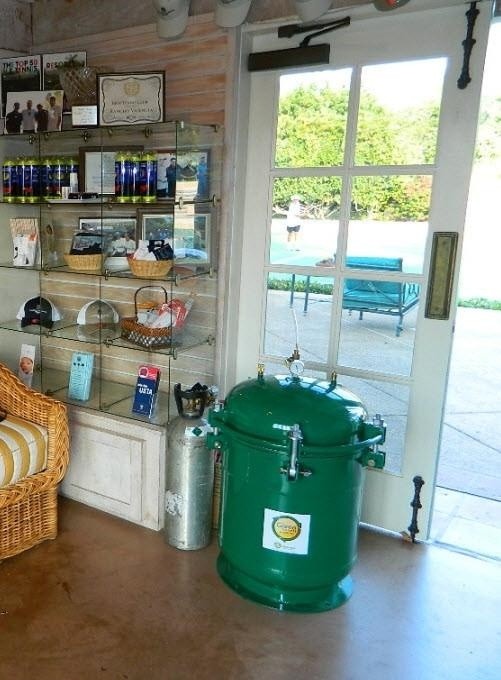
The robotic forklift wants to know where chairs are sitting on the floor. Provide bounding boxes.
[0,363,69,561]
[343,256,420,337]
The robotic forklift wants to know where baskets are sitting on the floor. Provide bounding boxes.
[127,255,173,277]
[119,316,182,349]
[63,253,105,271]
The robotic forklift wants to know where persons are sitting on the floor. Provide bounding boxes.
[285,196,303,249]
[6,96,62,135]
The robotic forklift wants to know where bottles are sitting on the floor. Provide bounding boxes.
[1,155,79,203]
[114,150,158,203]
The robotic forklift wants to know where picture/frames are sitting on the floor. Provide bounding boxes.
[78,212,211,265]
[96,70,167,128]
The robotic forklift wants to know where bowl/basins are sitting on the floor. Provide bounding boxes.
[58,65,114,108]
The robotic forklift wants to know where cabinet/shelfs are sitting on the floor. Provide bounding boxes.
[2,119,227,532]
